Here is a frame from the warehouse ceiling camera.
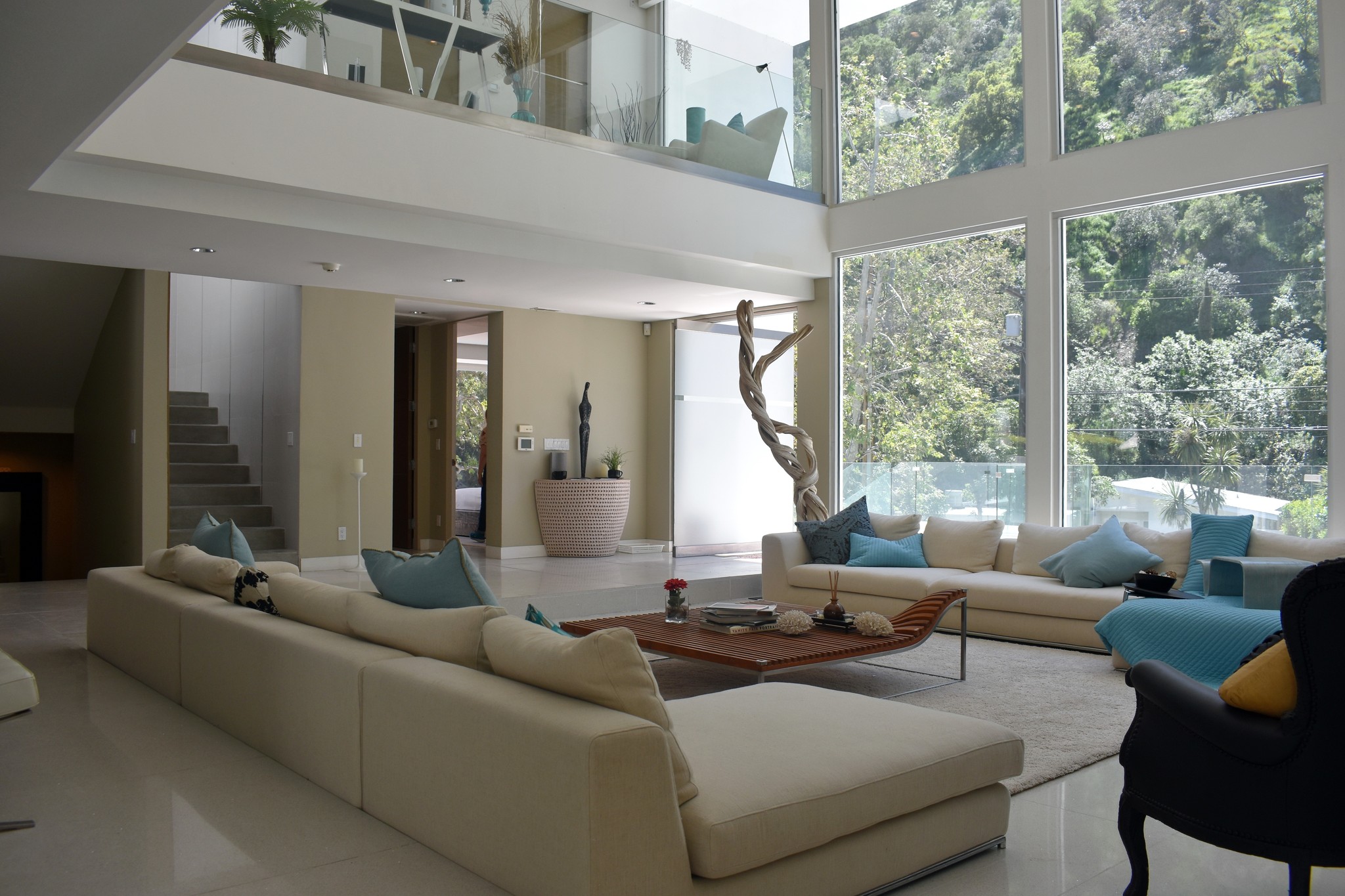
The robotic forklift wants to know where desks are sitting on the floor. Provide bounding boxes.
[535,477,630,556]
[315,0,505,113]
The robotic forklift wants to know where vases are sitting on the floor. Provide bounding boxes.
[664,595,689,623]
[510,88,537,124]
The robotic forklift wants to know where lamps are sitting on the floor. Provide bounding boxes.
[756,62,797,188]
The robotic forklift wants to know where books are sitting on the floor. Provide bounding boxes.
[699,603,781,634]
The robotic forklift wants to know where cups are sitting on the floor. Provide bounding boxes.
[815,610,824,618]
[843,614,854,621]
[552,471,567,480]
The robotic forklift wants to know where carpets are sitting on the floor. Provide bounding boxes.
[649,633,1138,798]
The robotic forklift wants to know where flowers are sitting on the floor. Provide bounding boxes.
[664,577,688,608]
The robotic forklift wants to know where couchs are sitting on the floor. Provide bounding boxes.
[85,532,1345,896]
[626,107,787,180]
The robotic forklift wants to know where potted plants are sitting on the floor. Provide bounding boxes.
[599,446,633,478]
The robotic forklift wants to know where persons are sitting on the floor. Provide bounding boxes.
[470,409,487,542]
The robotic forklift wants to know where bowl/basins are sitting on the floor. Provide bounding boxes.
[1133,573,1177,593]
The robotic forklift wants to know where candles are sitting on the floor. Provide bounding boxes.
[353,458,364,472]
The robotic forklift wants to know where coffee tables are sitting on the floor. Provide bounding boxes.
[559,586,968,699]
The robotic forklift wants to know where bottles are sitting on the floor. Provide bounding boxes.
[824,598,845,620]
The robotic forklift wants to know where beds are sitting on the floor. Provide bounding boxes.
[455,487,482,536]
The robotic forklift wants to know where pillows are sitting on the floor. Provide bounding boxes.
[795,495,876,565]
[1123,522,1192,590]
[1219,637,1299,716]
[921,516,1008,573]
[1011,522,1105,577]
[1247,529,1345,563]
[1179,512,1255,593]
[867,511,922,541]
[845,532,929,568]
[141,510,695,806]
[1039,515,1164,587]
